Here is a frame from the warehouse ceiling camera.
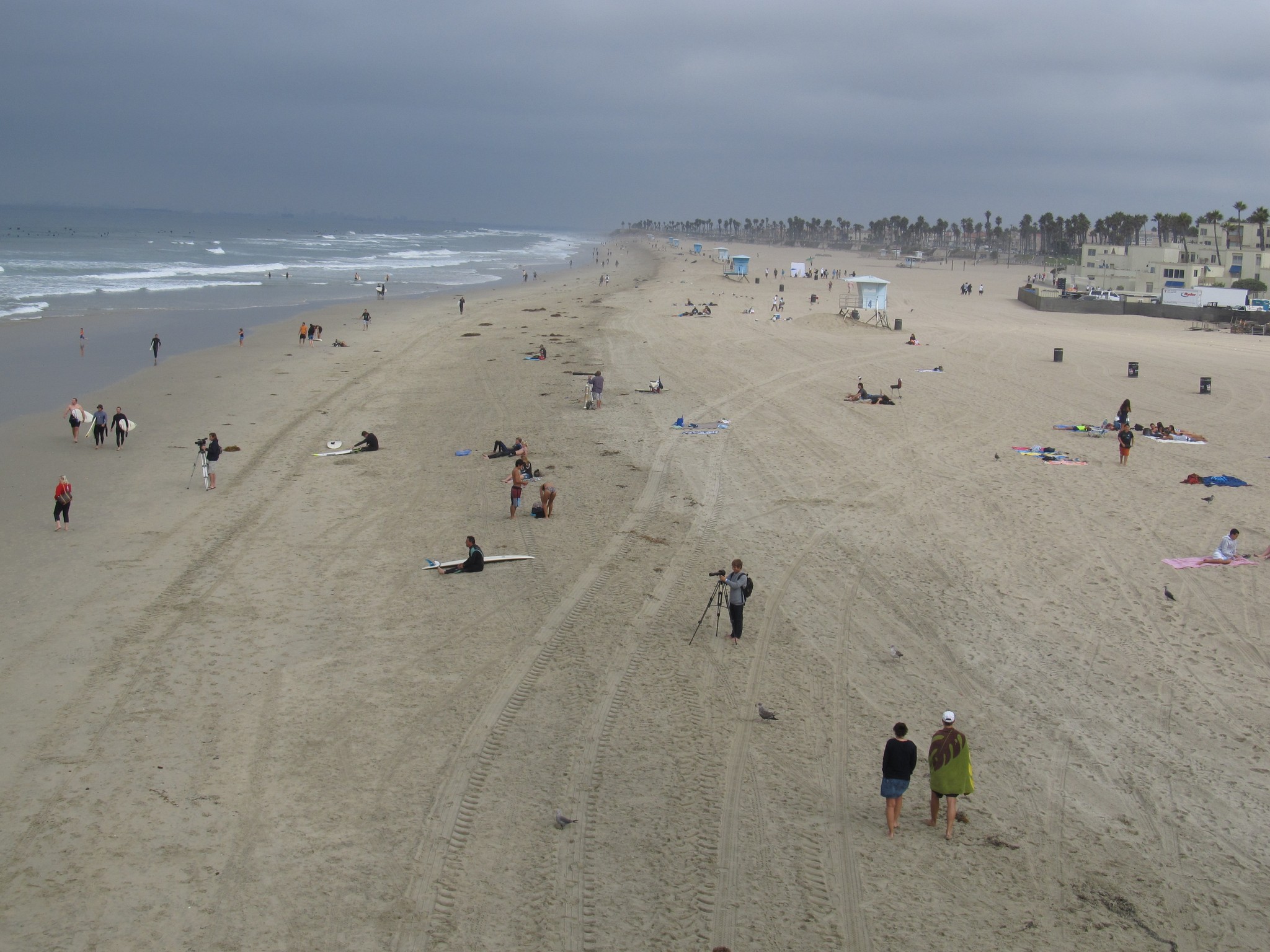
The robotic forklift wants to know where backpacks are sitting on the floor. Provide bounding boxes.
[942,711,955,723]
[217,446,222,454]
[731,572,753,603]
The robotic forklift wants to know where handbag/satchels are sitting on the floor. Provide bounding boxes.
[57,491,73,507]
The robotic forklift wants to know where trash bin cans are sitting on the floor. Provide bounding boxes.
[852,310,858,320]
[1053,348,1064,362]
[1128,362,1139,378]
[1200,377,1212,395]
[810,294,816,302]
[680,247,734,269]
[894,319,902,331]
[779,285,784,292]
[755,278,760,284]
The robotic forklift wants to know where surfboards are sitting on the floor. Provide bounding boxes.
[375,287,387,292]
[308,338,323,342]
[420,555,535,570]
[569,247,624,265]
[72,408,94,423]
[311,447,361,456]
[119,419,138,431]
[85,416,97,438]
[457,301,461,306]
[149,341,154,351]
[264,273,387,281]
[635,388,671,392]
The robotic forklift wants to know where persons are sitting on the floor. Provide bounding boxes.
[1149,421,1208,442]
[847,383,868,400]
[297,321,308,345]
[530,344,547,360]
[268,272,271,277]
[1053,425,1092,432]
[854,394,889,405]
[1117,423,1134,467]
[921,710,975,840]
[720,559,747,642]
[349,430,379,452]
[92,404,108,449]
[519,227,1116,322]
[910,334,920,345]
[490,438,523,452]
[78,328,88,342]
[437,535,484,574]
[482,441,528,461]
[286,272,289,278]
[1118,399,1131,434]
[313,325,322,339]
[361,309,371,330]
[1196,527,1240,565]
[460,296,465,313]
[588,370,604,408]
[202,433,219,490]
[880,722,917,838]
[354,272,389,297]
[110,407,128,451]
[1253,545,1270,560]
[509,459,528,520]
[500,454,533,485]
[238,328,244,347]
[308,324,314,347]
[539,482,556,519]
[53,475,72,531]
[63,398,85,443]
[151,334,161,360]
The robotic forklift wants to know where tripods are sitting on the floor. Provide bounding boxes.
[185,448,211,491]
[572,378,594,409]
[689,580,738,646]
